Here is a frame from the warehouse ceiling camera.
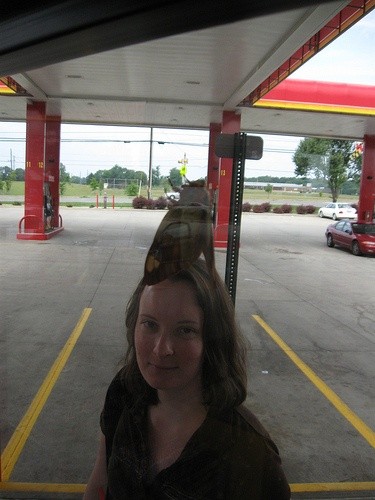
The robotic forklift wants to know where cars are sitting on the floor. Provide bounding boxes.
[325,219,375,256]
[318,202,357,221]
[166,191,180,199]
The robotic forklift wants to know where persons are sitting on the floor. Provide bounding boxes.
[82,255,292,500]
